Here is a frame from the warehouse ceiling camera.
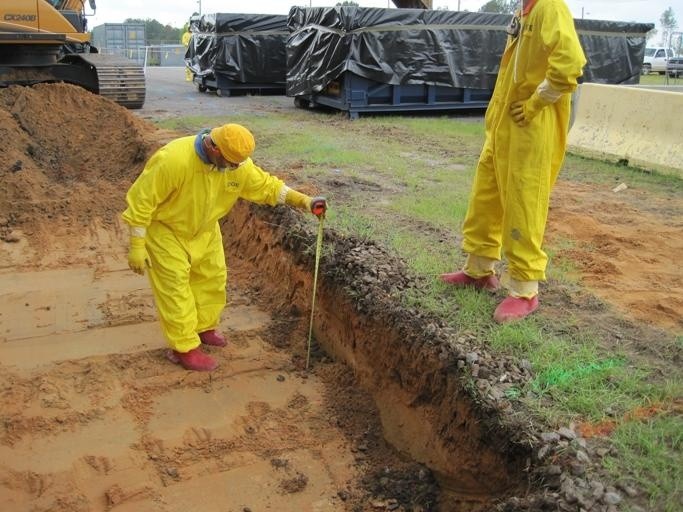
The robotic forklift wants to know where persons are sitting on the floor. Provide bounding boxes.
[118,122,331,371]
[180,23,194,83]
[435,0,587,328]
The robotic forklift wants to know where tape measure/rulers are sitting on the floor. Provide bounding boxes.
[306,201,327,370]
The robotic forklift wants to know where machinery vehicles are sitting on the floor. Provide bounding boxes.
[0,0,146,109]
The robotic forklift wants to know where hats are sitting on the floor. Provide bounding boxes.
[210,124,255,164]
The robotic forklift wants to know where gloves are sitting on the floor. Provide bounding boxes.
[281,187,329,215]
[129,228,152,275]
[510,82,564,128]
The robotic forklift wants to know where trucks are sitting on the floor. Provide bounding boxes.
[93,23,147,67]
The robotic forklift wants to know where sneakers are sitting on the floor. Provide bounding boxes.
[493,293,540,324]
[199,329,228,347]
[172,348,219,371]
[441,271,500,290]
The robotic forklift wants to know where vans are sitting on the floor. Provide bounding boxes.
[640,46,683,78]
